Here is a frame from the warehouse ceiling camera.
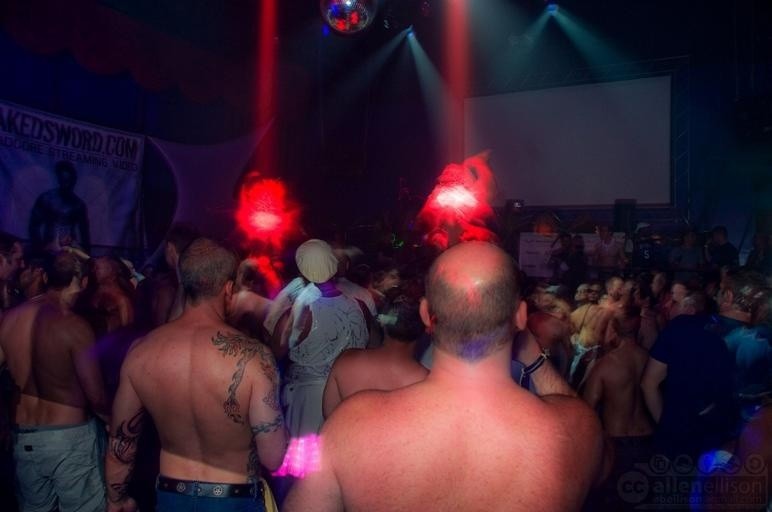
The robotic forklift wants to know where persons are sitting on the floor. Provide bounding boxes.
[639,266,772,512]
[322,288,430,419]
[282,240,616,512]
[582,308,650,512]
[0,253,113,512]
[0,226,738,395]
[29,160,91,258]
[289,238,375,438]
[106,237,288,512]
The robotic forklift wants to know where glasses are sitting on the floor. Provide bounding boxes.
[587,288,600,294]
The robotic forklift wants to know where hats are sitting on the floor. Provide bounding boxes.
[295,239,339,285]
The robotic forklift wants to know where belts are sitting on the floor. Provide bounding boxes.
[151,473,266,501]
